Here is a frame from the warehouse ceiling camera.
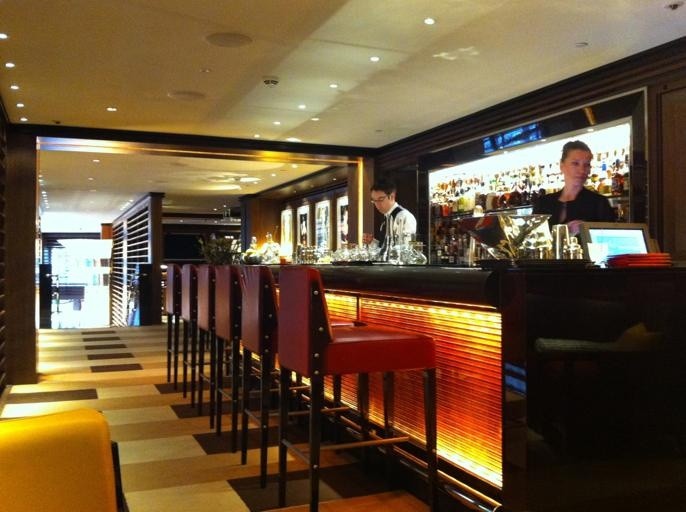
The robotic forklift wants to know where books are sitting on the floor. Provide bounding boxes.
[607,253,672,268]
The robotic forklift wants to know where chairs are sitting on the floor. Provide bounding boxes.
[237,264,368,492]
[212,265,339,454]
[166,265,229,391]
[276,264,441,512]
[180,264,270,409]
[197,264,301,431]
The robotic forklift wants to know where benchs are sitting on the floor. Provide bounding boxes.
[0,405,127,512]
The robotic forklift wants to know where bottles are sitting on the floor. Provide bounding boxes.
[293,245,316,265]
[429,148,631,222]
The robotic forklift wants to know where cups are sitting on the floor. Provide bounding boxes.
[331,242,426,265]
[521,219,582,258]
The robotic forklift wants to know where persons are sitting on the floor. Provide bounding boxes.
[258,231,280,257]
[340,207,349,243]
[361,178,417,247]
[531,141,614,245]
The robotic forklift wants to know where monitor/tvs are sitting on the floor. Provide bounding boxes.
[582,222,655,266]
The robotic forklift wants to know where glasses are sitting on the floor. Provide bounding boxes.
[371,196,385,203]
